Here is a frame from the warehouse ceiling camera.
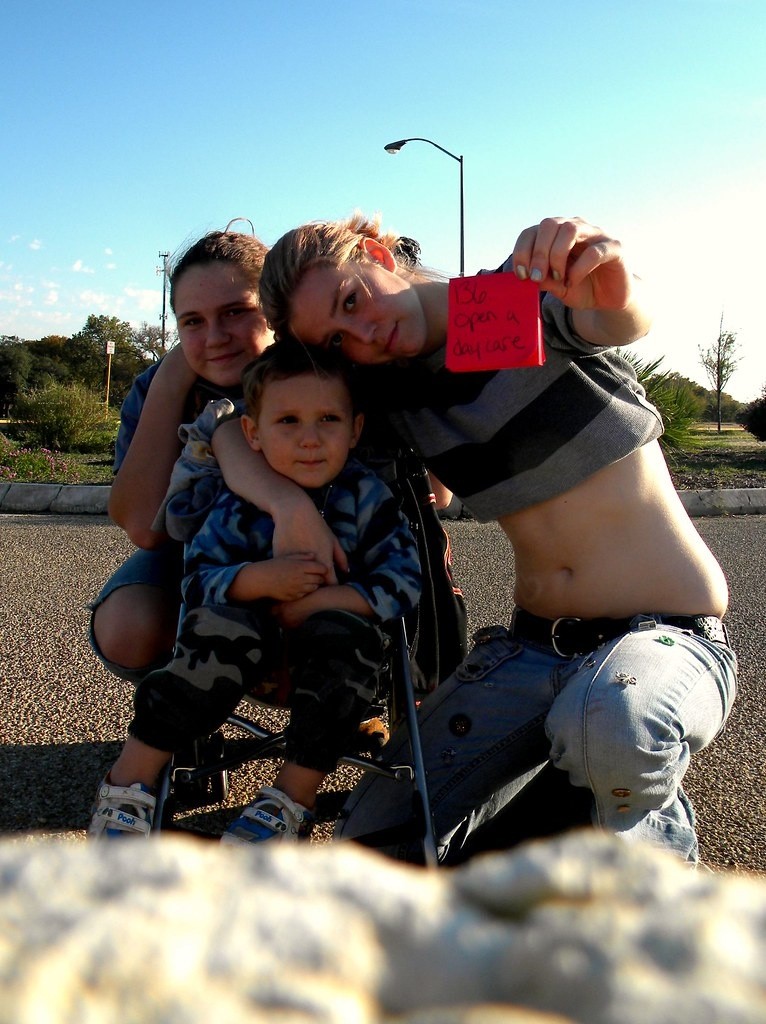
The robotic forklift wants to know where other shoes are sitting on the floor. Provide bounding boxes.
[346,716,390,749]
[215,789,315,853]
[92,774,158,849]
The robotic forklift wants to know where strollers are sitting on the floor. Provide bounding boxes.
[151,378,467,875]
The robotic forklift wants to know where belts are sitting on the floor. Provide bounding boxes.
[510,605,728,658]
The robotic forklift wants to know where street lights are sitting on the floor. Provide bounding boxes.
[155,251,170,361]
[384,137,466,274]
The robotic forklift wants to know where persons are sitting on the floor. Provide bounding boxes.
[212,216,749,872]
[89,229,458,756]
[88,338,426,849]
